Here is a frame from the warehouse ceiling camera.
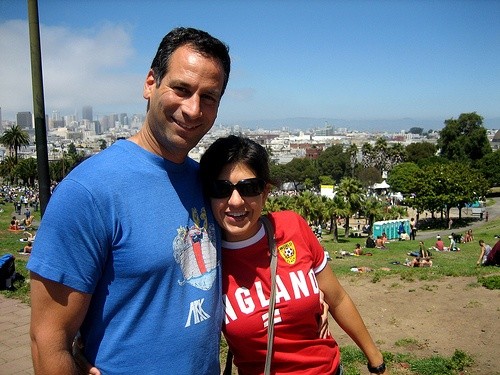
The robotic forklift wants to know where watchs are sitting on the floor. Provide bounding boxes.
[367,360,385,373]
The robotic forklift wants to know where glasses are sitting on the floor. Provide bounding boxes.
[202,178,268,199]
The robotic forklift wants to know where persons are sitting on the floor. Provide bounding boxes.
[199,135,390,375]
[25,27,230,375]
[0,179,500,281]
